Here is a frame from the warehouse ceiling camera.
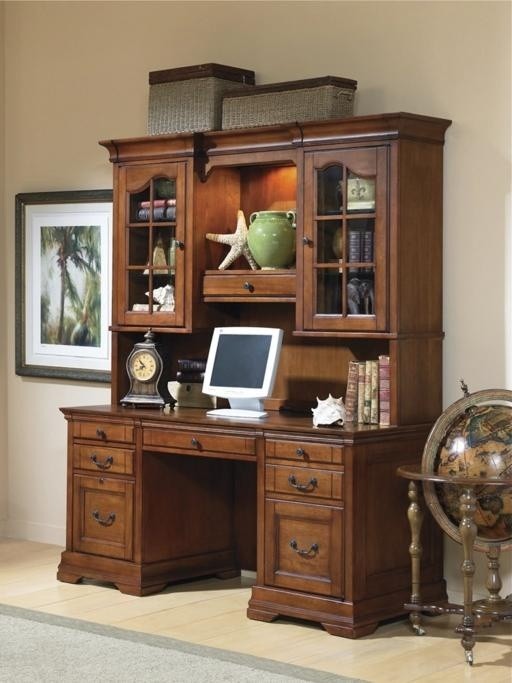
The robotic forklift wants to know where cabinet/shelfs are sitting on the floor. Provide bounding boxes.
[98,111,453,427]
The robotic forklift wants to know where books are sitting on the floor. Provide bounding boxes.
[132,198,175,221]
[177,358,206,382]
[132,303,175,312]
[339,200,373,272]
[345,353,391,426]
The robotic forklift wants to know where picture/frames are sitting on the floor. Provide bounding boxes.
[14,190,114,384]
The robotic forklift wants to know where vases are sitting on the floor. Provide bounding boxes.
[246,209,297,270]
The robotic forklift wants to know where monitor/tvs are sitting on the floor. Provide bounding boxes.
[201,327,284,418]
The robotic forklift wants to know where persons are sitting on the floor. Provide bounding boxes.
[347,277,375,314]
[71,309,95,346]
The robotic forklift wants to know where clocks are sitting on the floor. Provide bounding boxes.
[119,326,178,412]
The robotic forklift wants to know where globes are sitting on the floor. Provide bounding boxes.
[397,378,512,665]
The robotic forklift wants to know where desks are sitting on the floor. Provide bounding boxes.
[55,406,449,640]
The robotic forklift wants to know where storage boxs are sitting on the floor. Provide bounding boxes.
[144,62,358,136]
